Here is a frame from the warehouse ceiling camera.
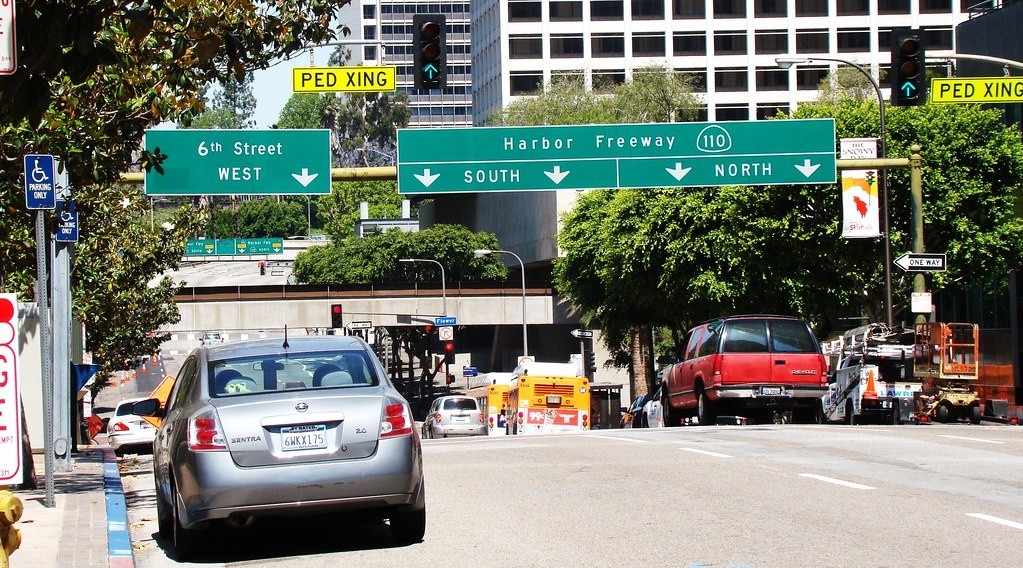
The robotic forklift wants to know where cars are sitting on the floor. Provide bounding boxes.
[102,397,163,454]
[131,339,427,540]
[199,333,224,347]
[620,314,833,429]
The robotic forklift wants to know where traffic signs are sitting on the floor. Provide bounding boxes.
[396,118,836,196]
[186,238,284,256]
[571,329,594,339]
[142,128,336,197]
[346,320,373,332]
[892,253,949,272]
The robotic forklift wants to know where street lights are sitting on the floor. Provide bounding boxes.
[776,56,893,332]
[399,259,451,396]
[472,249,527,357]
[150,197,167,233]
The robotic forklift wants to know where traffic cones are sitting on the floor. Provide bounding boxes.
[863,370,879,400]
[110,353,158,388]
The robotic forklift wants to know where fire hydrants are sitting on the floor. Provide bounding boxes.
[0,491,26,568]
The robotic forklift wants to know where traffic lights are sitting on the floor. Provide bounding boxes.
[259,261,266,275]
[588,351,598,373]
[331,304,344,329]
[447,375,456,384]
[889,27,931,106]
[412,13,447,92]
[445,340,456,365]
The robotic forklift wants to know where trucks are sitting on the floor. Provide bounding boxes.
[817,322,983,426]
[421,362,591,438]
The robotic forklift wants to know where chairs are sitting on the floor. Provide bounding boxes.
[321,372,352,386]
[227,380,258,391]
[312,364,343,387]
